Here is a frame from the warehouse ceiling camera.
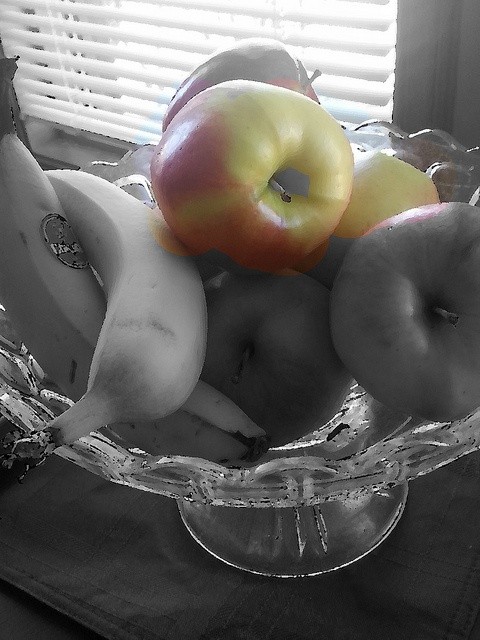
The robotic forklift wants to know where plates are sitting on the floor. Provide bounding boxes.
[0,119,479,579]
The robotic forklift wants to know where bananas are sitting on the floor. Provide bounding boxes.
[0,55,267,463]
[11,168,208,467]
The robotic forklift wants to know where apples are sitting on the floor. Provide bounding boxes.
[198,266,355,449]
[329,201,480,424]
[307,150,440,287]
[152,78,356,273]
[162,43,322,132]
